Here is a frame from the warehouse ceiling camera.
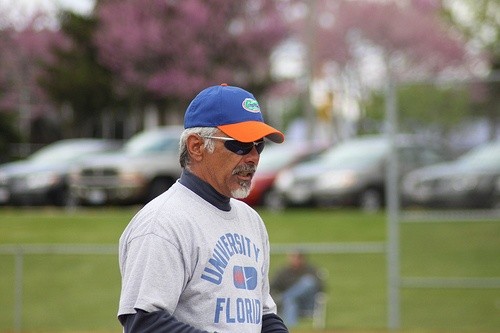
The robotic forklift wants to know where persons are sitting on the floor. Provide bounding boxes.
[117,84,288,333]
[269,250,324,322]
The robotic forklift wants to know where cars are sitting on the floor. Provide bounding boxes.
[68,124,186,208]
[0,139,120,206]
[396,135,499,207]
[272,134,463,212]
[244,136,344,208]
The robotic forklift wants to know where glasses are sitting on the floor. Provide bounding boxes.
[212,138,266,156]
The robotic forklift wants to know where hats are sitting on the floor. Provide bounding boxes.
[184,83,284,144]
